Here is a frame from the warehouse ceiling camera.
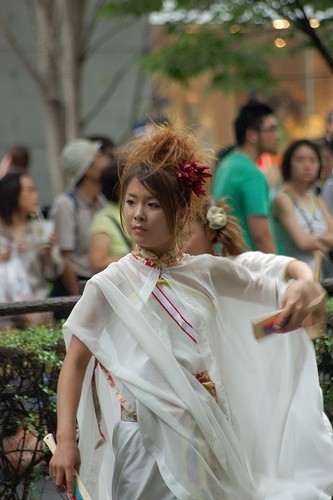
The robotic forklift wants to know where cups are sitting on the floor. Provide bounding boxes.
[35,221,55,247]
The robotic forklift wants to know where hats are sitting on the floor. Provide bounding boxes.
[59,139,103,191]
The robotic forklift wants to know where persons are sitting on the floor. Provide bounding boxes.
[48,126,333,500]
[211,99,333,298]
[182,197,314,281]
[0,134,134,481]
